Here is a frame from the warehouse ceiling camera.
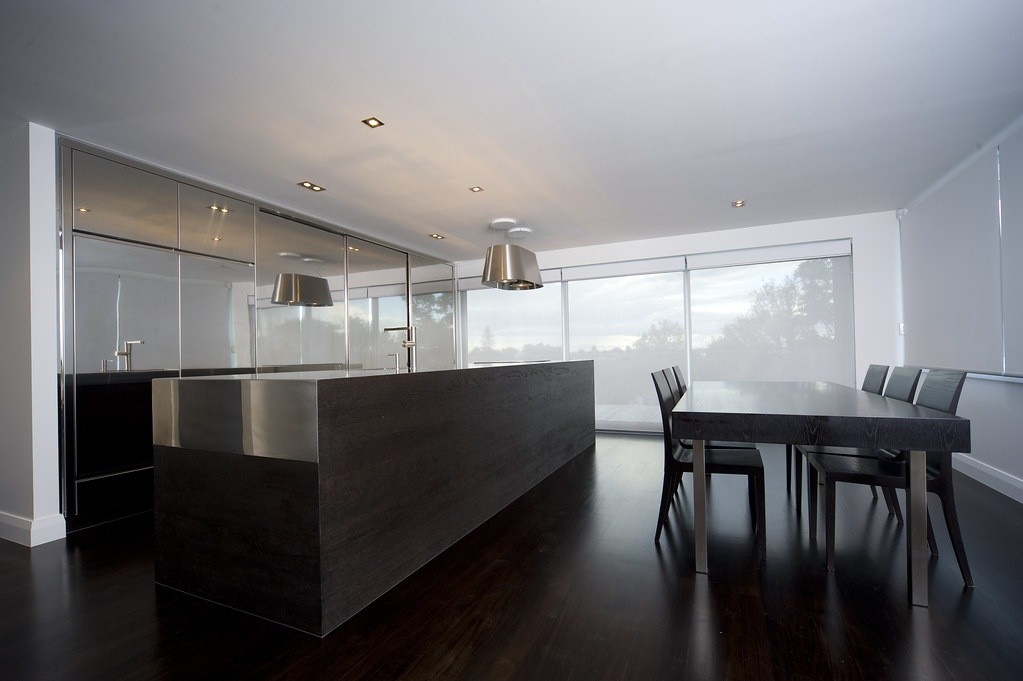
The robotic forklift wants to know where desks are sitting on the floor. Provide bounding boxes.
[671,380,972,607]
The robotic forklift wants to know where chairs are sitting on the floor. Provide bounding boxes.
[786,364,975,590]
[651,365,768,549]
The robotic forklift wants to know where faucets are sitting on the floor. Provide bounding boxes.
[115,340,145,371]
[384,326,417,370]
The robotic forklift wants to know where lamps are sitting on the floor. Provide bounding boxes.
[481,219,544,291]
[272,253,335,308]
